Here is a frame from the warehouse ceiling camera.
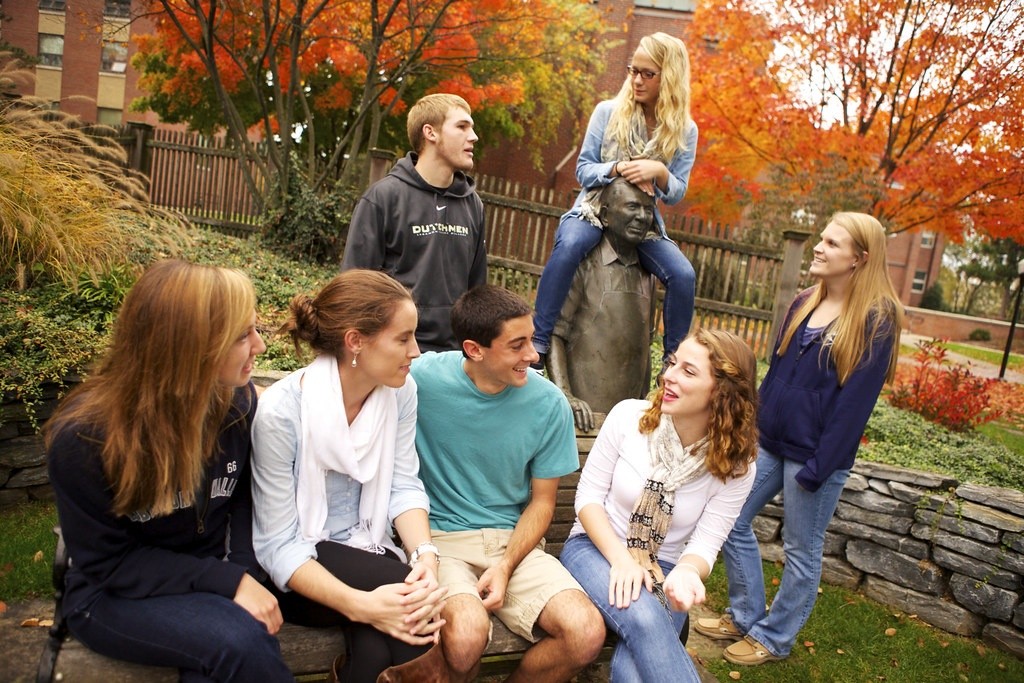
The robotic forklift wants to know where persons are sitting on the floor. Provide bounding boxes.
[250,270,449,683]
[410,286,606,683]
[342,93,488,353]
[559,326,758,683]
[695,210,904,666]
[531,31,696,389]
[546,177,657,433]
[47,259,294,683]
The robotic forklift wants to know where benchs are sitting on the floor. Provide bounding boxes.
[34,411,689,683]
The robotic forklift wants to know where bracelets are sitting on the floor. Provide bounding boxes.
[615,160,623,177]
[677,563,700,575]
[409,543,440,569]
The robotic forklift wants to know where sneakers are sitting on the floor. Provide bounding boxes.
[693,613,746,641]
[723,633,782,666]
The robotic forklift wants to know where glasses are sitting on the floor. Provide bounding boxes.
[626,65,664,80]
